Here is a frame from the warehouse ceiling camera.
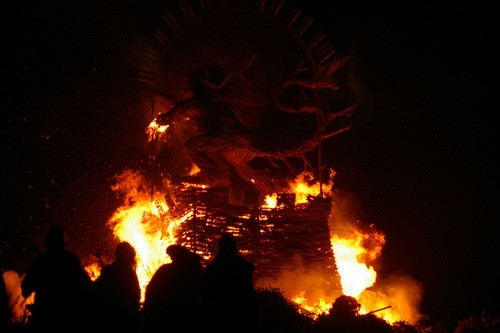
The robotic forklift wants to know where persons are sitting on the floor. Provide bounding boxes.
[20,225,93,333]
[144,244,209,333]
[89,241,143,333]
[203,232,260,333]
